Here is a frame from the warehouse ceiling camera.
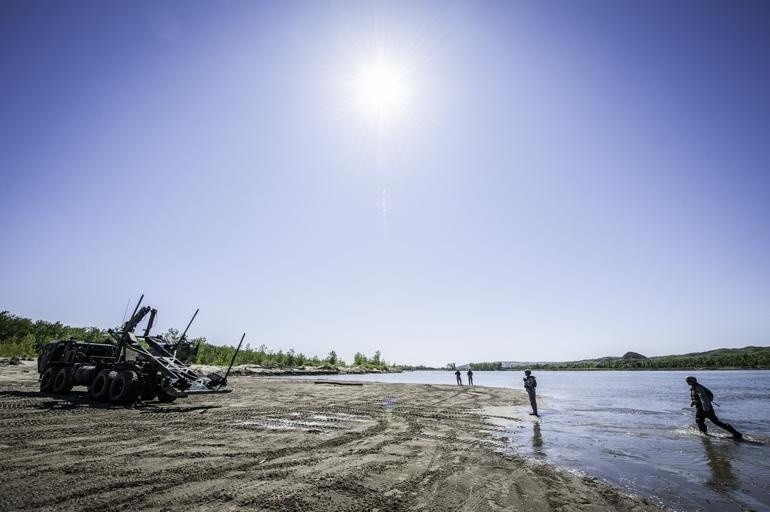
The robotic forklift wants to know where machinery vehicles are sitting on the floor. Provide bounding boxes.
[38,294,245,405]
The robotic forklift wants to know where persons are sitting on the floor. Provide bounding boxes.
[455,370,463,386]
[524,368,540,417]
[468,368,473,386]
[686,376,743,439]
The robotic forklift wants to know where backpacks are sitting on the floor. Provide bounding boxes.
[694,384,713,401]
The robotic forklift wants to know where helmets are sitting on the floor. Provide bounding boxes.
[525,370,531,374]
